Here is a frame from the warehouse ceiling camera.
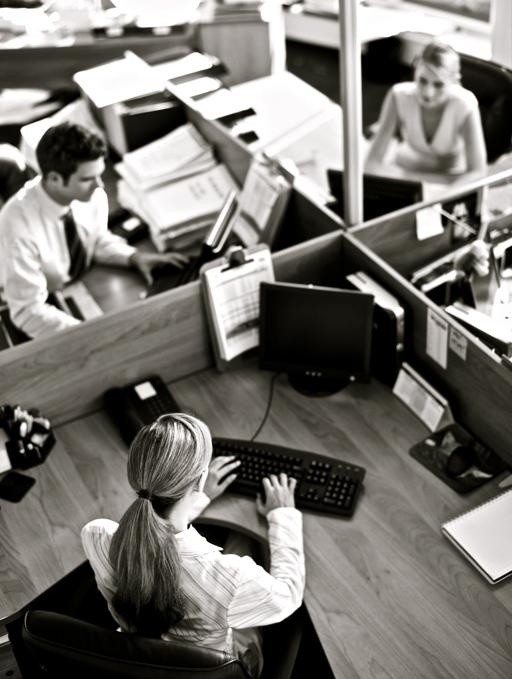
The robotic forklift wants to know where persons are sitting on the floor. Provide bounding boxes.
[80,412,306,679]
[0,121,189,340]
[363,40,488,185]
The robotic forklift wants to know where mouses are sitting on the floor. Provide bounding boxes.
[445,444,475,477]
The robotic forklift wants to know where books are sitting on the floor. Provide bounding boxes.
[440,485,512,585]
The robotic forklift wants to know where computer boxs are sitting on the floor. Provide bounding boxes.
[345,270,417,386]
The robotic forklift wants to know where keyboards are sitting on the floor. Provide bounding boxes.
[146,255,200,297]
[212,437,366,518]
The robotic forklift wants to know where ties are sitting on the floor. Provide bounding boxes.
[62,210,86,279]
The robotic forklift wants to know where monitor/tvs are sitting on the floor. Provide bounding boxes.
[199,191,241,259]
[326,171,423,222]
[258,282,374,399]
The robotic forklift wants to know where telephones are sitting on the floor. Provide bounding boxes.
[103,374,181,450]
